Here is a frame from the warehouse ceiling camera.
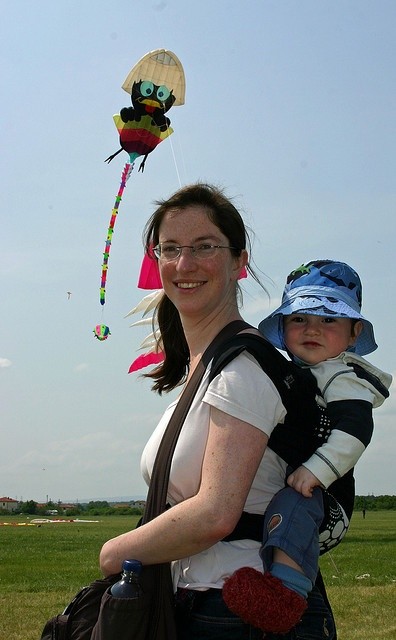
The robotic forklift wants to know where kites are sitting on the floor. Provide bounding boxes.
[91,46,188,342]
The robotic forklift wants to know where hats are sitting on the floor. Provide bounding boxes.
[258,260,378,356]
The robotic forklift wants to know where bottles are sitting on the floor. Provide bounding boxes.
[109,558,144,599]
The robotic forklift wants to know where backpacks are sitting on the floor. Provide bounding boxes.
[209,332,355,556]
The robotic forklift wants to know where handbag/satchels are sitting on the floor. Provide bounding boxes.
[40,559,258,640]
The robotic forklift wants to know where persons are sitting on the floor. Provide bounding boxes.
[221,258,392,634]
[99,183,337,640]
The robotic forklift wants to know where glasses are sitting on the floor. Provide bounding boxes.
[152,241,238,262]
